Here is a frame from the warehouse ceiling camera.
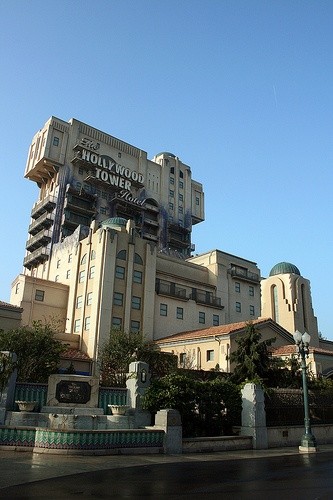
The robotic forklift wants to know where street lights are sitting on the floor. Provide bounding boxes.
[293,329,320,453]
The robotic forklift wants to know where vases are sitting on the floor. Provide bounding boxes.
[16,400,39,412]
[108,404,131,415]
